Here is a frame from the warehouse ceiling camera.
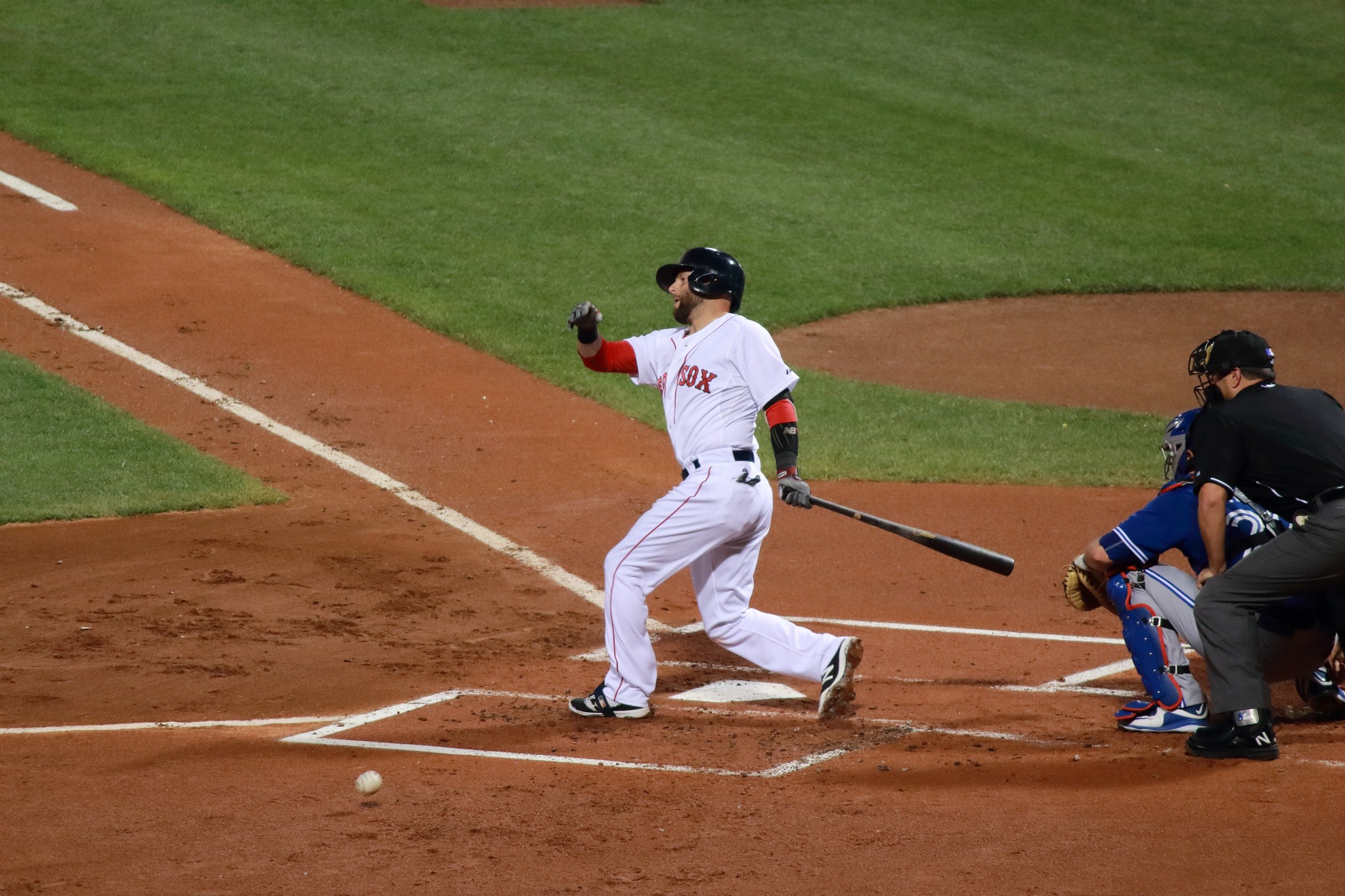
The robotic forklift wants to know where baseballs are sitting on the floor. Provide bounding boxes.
[354,770,383,795]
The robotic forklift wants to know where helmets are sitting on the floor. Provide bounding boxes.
[1160,409,1201,481]
[655,247,744,312]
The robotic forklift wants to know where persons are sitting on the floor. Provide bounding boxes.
[1185,330,1345,761]
[567,247,864,720]
[1074,407,1345,735]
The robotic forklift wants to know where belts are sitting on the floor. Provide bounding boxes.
[1306,488,1345,513]
[681,450,754,481]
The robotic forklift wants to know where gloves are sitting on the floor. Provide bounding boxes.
[736,467,760,486]
[567,301,603,335]
[776,466,813,509]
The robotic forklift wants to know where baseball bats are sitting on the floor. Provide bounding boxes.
[780,485,1015,577]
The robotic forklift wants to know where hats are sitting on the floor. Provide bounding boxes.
[1194,329,1274,371]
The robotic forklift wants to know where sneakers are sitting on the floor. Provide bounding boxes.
[817,636,864,719]
[1115,702,1209,732]
[568,681,651,719]
[1184,719,1279,761]
[1296,667,1345,717]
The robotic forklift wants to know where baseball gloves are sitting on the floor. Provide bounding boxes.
[1065,551,1120,617]
